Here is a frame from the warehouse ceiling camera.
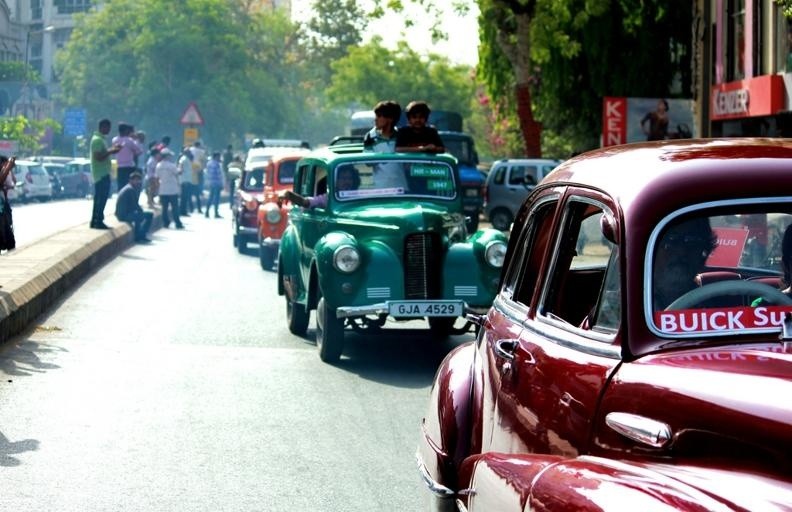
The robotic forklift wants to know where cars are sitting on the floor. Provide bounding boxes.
[228,138,313,271]
[7,148,91,207]
[277,140,511,364]
[414,136,791,512]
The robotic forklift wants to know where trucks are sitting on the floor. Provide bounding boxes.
[350,108,485,235]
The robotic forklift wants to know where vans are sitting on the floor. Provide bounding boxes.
[477,162,500,187]
[482,158,566,231]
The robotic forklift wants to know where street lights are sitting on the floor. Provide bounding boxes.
[18,25,56,158]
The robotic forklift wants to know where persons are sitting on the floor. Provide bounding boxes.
[277,163,360,211]
[361,100,402,153]
[640,98,670,141]
[576,213,716,329]
[87,117,242,243]
[394,101,446,153]
[750,223,792,309]
[1,154,16,251]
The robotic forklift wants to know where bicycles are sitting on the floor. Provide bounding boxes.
[738,223,784,271]
